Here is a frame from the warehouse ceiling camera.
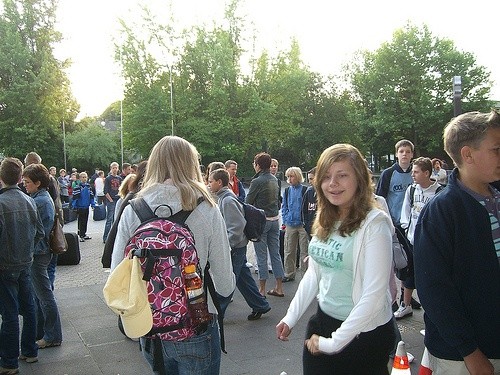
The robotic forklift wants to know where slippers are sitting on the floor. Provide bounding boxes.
[267,289,284,297]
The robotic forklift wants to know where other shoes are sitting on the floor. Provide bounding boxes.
[35,338,61,349]
[248,307,271,320]
[83,236,92,239]
[282,277,295,282]
[19,348,38,363]
[393,301,413,320]
[80,237,85,242]
[400,294,421,309]
[0,366,20,375]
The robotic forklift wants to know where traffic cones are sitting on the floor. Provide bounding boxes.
[418,346,433,375]
[390,341,412,375]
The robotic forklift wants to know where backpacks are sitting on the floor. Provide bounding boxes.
[124,196,209,341]
[222,193,266,242]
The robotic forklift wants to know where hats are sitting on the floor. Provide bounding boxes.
[103,248,153,338]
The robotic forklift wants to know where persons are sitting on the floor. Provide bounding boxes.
[412,111,500,375]
[200,153,318,321]
[0,152,63,375]
[49,161,147,243]
[371,140,457,320]
[109,135,236,375]
[276,144,396,375]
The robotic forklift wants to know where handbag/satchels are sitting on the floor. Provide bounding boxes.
[93,203,106,221]
[57,232,81,266]
[49,214,68,257]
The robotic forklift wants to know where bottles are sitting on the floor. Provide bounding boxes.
[184,264,209,323]
